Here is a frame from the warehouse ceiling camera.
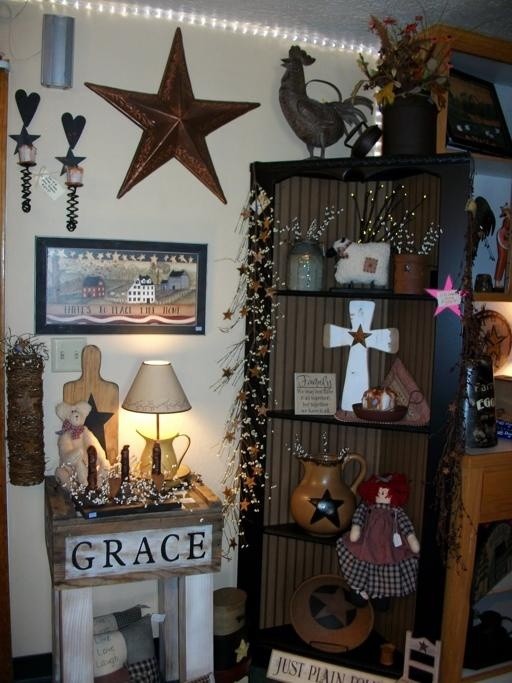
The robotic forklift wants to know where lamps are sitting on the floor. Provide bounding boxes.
[121,356,194,487]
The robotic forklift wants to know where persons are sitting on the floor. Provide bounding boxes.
[336,471,420,610]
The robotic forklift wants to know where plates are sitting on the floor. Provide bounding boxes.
[132,464,192,489]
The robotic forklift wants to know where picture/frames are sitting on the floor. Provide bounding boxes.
[444,64,512,162]
[31,231,210,337]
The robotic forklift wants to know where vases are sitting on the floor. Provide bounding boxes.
[376,94,439,157]
[285,237,326,291]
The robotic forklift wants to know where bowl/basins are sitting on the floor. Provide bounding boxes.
[291,574,375,652]
[363,386,398,412]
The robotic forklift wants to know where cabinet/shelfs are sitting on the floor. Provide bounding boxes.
[239,148,478,683]
[43,468,225,682]
[407,20,511,682]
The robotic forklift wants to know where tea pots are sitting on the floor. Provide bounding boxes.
[135,429,191,478]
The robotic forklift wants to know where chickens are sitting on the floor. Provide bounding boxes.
[279,46,375,160]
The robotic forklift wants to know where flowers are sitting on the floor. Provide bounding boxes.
[333,0,496,113]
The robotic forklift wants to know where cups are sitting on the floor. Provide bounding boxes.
[473,273,493,292]
[380,644,396,665]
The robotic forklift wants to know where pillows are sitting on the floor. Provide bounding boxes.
[89,611,162,682]
[88,604,143,635]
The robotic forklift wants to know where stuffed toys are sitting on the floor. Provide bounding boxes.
[57,400,124,488]
